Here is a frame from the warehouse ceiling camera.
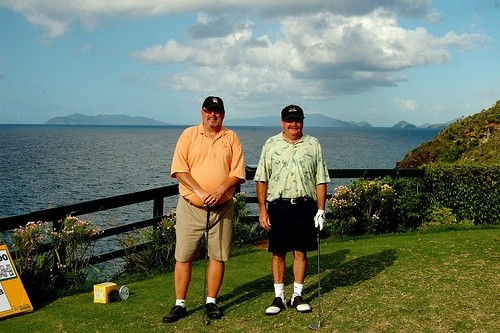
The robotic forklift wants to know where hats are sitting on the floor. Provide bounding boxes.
[281,105,305,119]
[203,96,223,111]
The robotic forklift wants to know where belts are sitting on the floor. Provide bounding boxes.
[269,198,315,205]
[183,197,231,212]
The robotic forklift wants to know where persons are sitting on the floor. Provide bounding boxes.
[162,96,246,323]
[252,105,331,315]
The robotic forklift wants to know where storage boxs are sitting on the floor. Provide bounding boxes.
[94,282,118,305]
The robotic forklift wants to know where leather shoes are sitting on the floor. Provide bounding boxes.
[291,296,311,312]
[205,303,222,319]
[163,306,186,323]
[265,297,286,315]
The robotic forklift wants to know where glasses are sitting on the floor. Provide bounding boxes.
[203,107,223,114]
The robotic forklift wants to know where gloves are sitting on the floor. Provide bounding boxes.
[314,210,325,231]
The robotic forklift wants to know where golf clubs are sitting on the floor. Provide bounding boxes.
[203,202,211,326]
[309,225,322,331]
